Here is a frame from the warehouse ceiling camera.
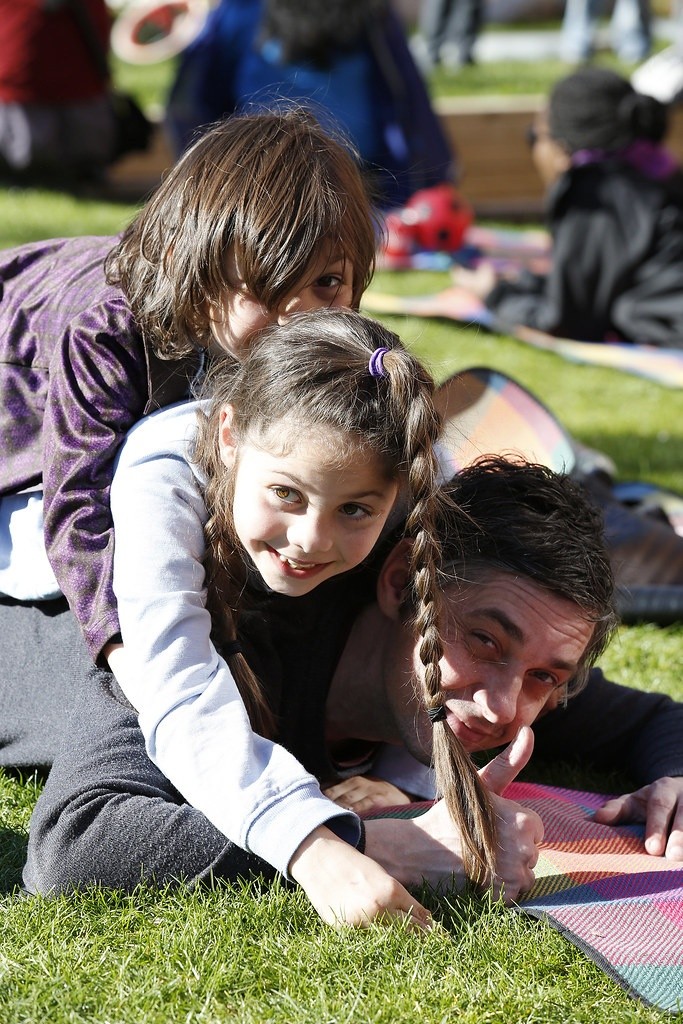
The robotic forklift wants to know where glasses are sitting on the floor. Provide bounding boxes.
[525,128,555,149]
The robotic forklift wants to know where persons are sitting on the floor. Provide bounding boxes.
[558,0,648,66]
[0,310,434,947]
[420,0,489,73]
[452,60,683,349]
[0,1,155,191]
[0,450,683,911]
[0,103,388,712]
[167,0,459,212]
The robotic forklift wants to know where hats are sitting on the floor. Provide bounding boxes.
[628,43,683,104]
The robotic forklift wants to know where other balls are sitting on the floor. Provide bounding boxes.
[407,189,467,252]
